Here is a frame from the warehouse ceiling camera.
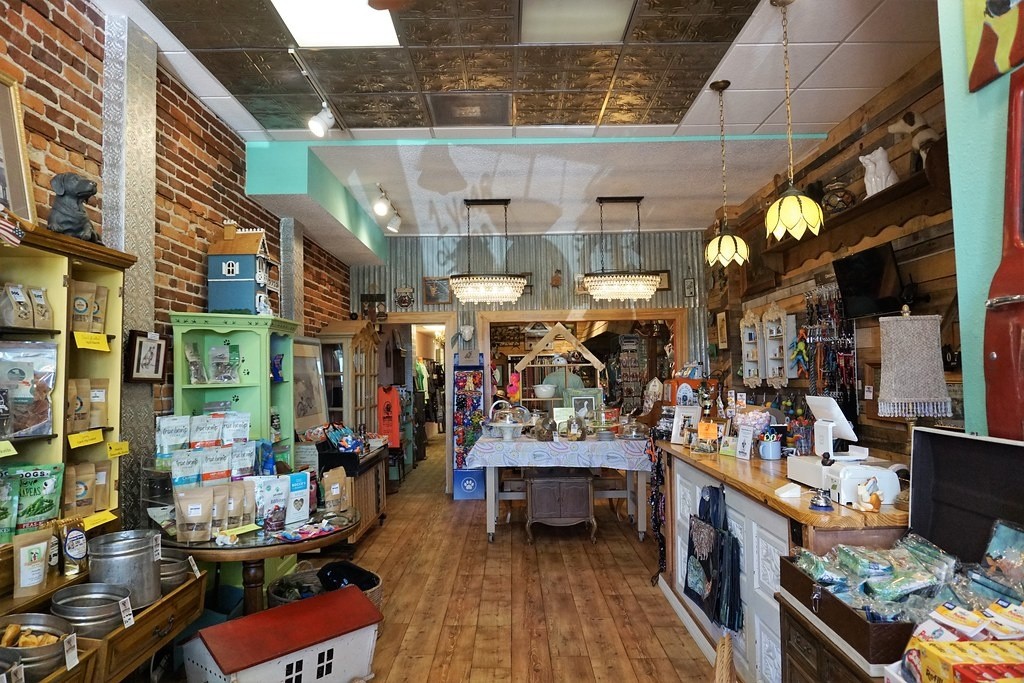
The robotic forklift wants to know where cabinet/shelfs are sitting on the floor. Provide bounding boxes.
[168,312,301,485]
[0,214,209,683]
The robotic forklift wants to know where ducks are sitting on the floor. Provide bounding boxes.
[578,401,589,419]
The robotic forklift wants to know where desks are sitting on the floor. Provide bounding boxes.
[656,438,909,682]
[464,434,654,544]
[772,592,883,683]
[154,505,362,619]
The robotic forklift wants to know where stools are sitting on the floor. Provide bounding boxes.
[523,469,599,544]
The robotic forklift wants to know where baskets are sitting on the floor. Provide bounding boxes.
[478,400,525,436]
[709,633,740,683]
[266,567,384,641]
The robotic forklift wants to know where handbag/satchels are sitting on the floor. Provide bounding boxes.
[682,485,745,632]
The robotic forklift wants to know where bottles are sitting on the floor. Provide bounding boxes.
[535,417,557,442]
[567,417,586,441]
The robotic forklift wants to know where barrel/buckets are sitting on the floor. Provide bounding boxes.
[0,530,189,683]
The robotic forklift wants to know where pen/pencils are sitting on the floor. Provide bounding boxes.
[760,434,764,441]
[777,434,783,441]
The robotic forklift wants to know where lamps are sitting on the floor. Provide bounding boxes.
[450,198,526,304]
[766,0,826,241]
[705,80,751,266]
[375,197,403,234]
[583,196,662,302]
[878,304,953,511]
[307,103,336,136]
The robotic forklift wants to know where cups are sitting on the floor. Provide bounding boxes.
[759,441,781,460]
[502,428,513,440]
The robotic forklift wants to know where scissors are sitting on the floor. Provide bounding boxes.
[763,425,777,441]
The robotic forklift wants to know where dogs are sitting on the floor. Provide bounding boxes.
[47,171,106,247]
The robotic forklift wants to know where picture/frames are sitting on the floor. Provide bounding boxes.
[653,270,672,291]
[124,328,168,382]
[294,335,330,434]
[422,276,453,304]
[0,72,39,226]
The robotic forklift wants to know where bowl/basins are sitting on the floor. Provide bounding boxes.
[531,385,558,398]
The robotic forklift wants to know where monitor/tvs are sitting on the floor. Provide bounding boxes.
[832,240,906,320]
